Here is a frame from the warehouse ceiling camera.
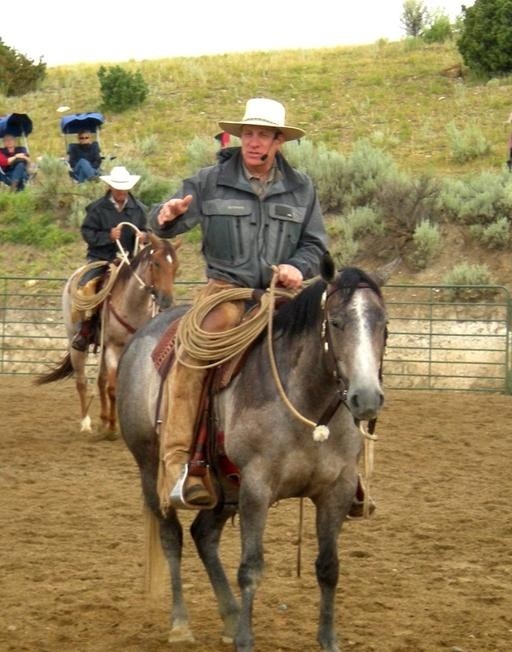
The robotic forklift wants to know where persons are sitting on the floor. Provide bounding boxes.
[146,96,331,505]
[0,134,31,192]
[71,166,149,352]
[64,130,102,182]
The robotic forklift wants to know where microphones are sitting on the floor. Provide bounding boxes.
[260,131,281,161]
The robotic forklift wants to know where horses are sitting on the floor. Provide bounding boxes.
[115,251,406,651]
[32,227,183,442]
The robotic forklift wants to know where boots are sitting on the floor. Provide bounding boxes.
[72,314,97,351]
[156,349,211,504]
[10,181,18,192]
[88,176,100,184]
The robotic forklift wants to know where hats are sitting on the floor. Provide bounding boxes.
[218,98,306,142]
[99,167,141,190]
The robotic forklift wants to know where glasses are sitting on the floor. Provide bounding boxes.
[79,137,89,139]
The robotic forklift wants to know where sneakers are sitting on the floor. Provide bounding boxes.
[347,474,376,517]
